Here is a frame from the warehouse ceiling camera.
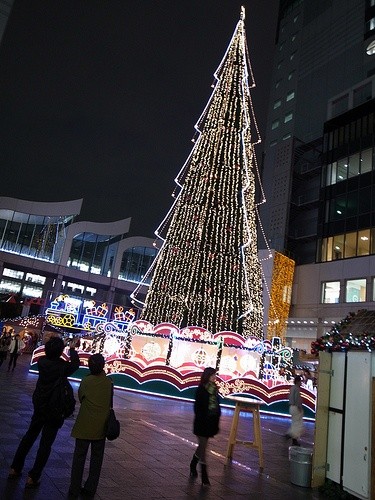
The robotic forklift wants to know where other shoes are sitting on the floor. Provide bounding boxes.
[26,476,41,487]
[8,468,24,477]
[292,437,301,446]
[69,485,97,498]
[286,433,292,439]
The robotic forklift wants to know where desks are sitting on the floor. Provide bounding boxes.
[224,395,267,471]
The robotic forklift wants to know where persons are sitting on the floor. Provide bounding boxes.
[188,367,221,487]
[275,367,314,389]
[0,328,26,373]
[69,353,121,500]
[11,335,80,489]
[283,375,304,447]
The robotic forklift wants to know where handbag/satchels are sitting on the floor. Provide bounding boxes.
[105,383,120,440]
[48,361,75,421]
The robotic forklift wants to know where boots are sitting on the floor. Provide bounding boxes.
[190,454,199,476]
[200,463,211,486]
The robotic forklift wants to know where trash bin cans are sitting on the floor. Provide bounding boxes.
[289,446,313,488]
